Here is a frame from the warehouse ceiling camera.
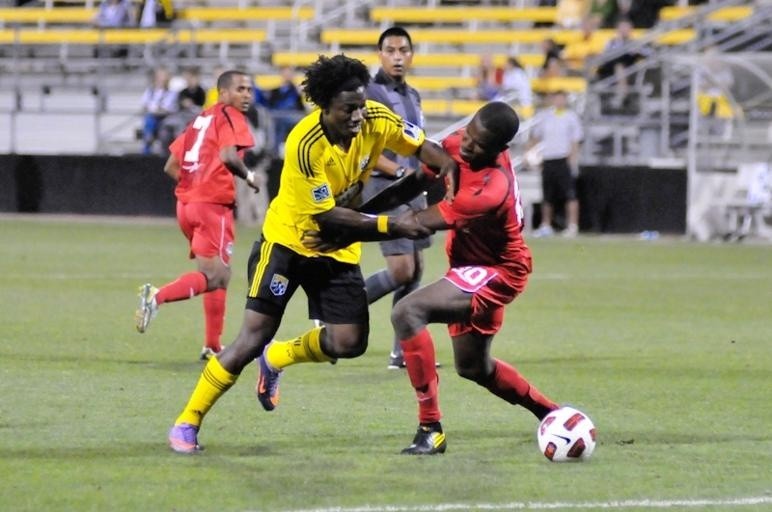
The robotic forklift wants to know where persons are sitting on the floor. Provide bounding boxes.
[603,1,647,29]
[315,27,442,371]
[522,90,583,239]
[234,104,277,222]
[500,57,528,100]
[136,0,176,28]
[599,21,654,107]
[301,101,560,455]
[268,65,306,141]
[90,0,129,95]
[136,67,177,158]
[169,66,196,91]
[537,38,568,78]
[161,84,203,141]
[134,70,261,360]
[172,55,459,455]
[542,51,559,75]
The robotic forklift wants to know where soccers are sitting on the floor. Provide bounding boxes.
[536,406,597,462]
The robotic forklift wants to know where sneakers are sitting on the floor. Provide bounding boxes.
[166,424,203,454]
[255,340,282,412]
[199,345,227,361]
[136,283,159,332]
[389,351,440,371]
[313,319,337,367]
[402,421,447,454]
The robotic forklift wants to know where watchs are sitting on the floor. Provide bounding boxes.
[392,166,406,177]
